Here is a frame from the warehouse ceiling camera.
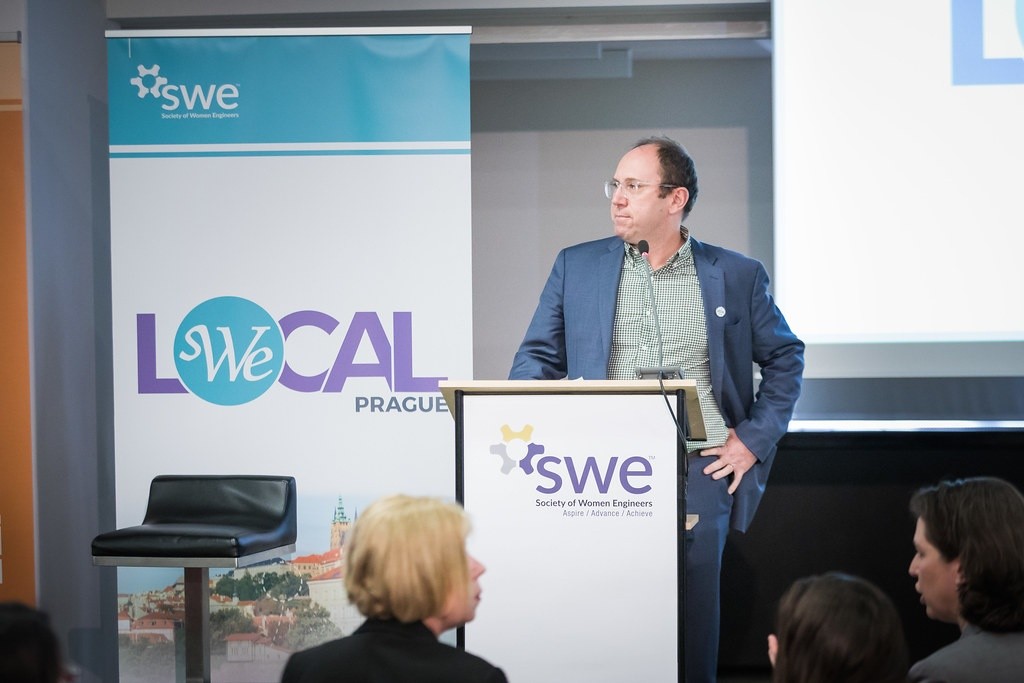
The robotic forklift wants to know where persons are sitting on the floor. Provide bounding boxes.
[280,494,508,683]
[902,478,1024,683]
[509,136,806,683]
[0,601,63,683]
[766,573,902,683]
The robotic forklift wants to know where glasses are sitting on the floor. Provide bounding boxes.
[604,179,680,199]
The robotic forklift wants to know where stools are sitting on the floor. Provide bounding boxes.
[93,475,297,683]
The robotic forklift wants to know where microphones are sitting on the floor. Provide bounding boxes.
[637,240,684,379]
